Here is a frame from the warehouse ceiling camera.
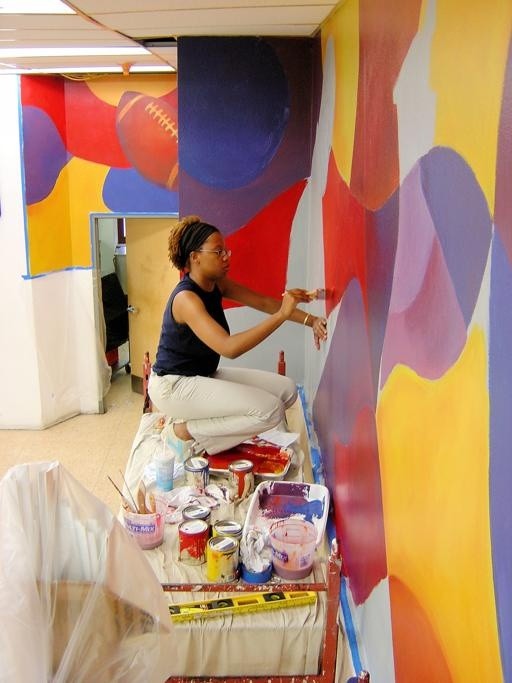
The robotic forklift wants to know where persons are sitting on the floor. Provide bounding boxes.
[147,217,328,465]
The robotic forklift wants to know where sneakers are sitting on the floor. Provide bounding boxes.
[160,422,198,465]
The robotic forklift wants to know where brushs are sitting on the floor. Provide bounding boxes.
[281,289,326,301]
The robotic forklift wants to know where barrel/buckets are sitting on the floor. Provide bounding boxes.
[268,518,318,580]
[121,494,166,550]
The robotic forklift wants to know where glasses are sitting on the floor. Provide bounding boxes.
[191,249,232,258]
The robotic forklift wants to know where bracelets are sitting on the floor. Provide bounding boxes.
[303,313,312,327]
